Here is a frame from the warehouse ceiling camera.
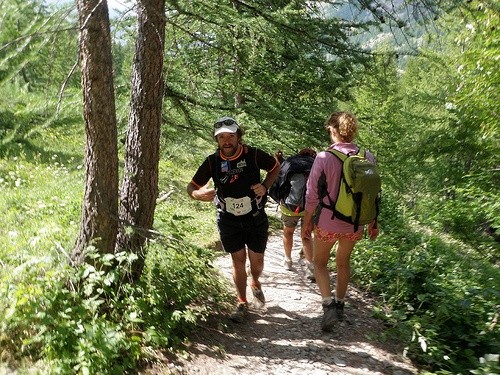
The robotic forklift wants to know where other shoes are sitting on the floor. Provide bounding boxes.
[333,298,344,321]
[281,257,293,270]
[304,263,317,281]
[321,300,340,333]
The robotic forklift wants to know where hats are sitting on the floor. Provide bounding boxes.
[214,116,240,136]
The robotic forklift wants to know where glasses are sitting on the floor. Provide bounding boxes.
[214,119,240,128]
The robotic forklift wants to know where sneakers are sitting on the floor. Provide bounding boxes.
[250,279,266,307]
[230,302,248,323]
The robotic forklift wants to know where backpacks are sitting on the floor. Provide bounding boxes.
[285,174,309,212]
[325,146,381,225]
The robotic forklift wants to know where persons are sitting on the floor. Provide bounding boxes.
[187,117,281,311]
[302,111,379,334]
[268,149,318,271]
